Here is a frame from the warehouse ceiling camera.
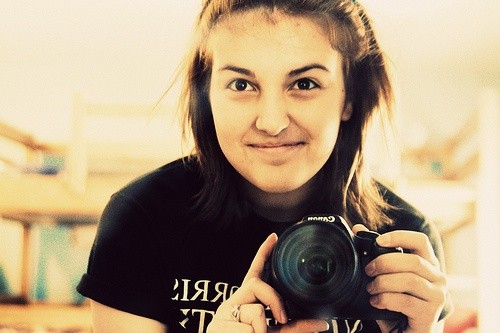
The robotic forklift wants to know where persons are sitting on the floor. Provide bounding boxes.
[75,0,445,333]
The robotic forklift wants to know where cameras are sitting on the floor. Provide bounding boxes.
[264,214,409,321]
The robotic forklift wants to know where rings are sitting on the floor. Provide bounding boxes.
[231,304,240,321]
